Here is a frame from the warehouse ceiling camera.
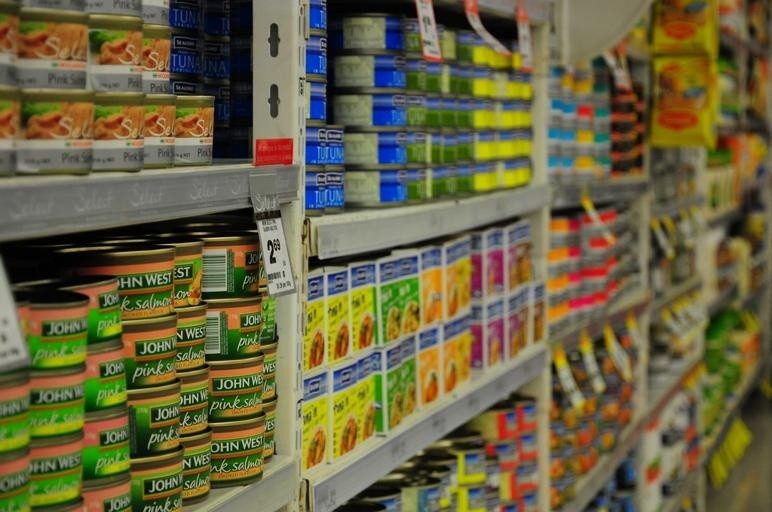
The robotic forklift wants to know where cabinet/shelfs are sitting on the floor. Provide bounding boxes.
[0,1,771,511]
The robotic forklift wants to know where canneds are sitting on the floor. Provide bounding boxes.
[0,218,280,511]
[169,1,346,217]
[303,216,545,471]
[331,11,535,208]
[334,393,538,512]
[1,1,216,177]
